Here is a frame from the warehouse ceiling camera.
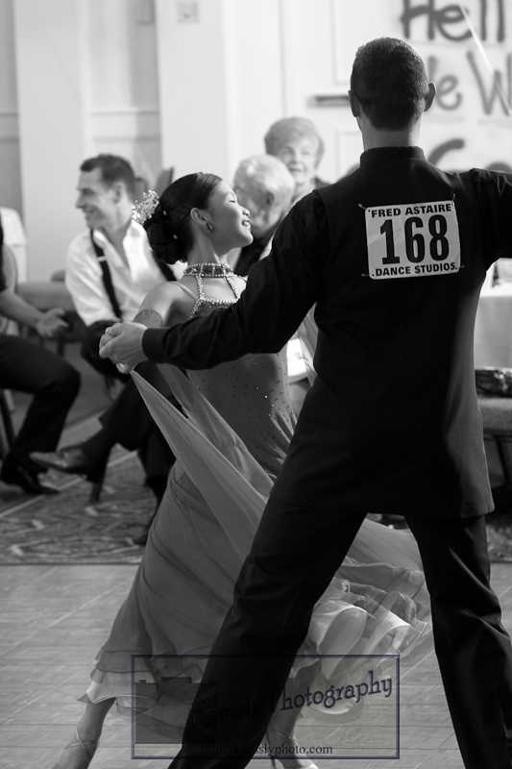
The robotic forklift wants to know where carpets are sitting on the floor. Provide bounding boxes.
[0,374,308,565]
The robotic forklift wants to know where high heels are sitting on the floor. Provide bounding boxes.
[49,729,98,769]
[263,733,318,768]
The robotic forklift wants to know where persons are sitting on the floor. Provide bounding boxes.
[97,37,511,769]
[52,171,435,769]
[0,117,343,495]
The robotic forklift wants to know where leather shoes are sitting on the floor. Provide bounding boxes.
[0,464,61,493]
[30,443,107,486]
